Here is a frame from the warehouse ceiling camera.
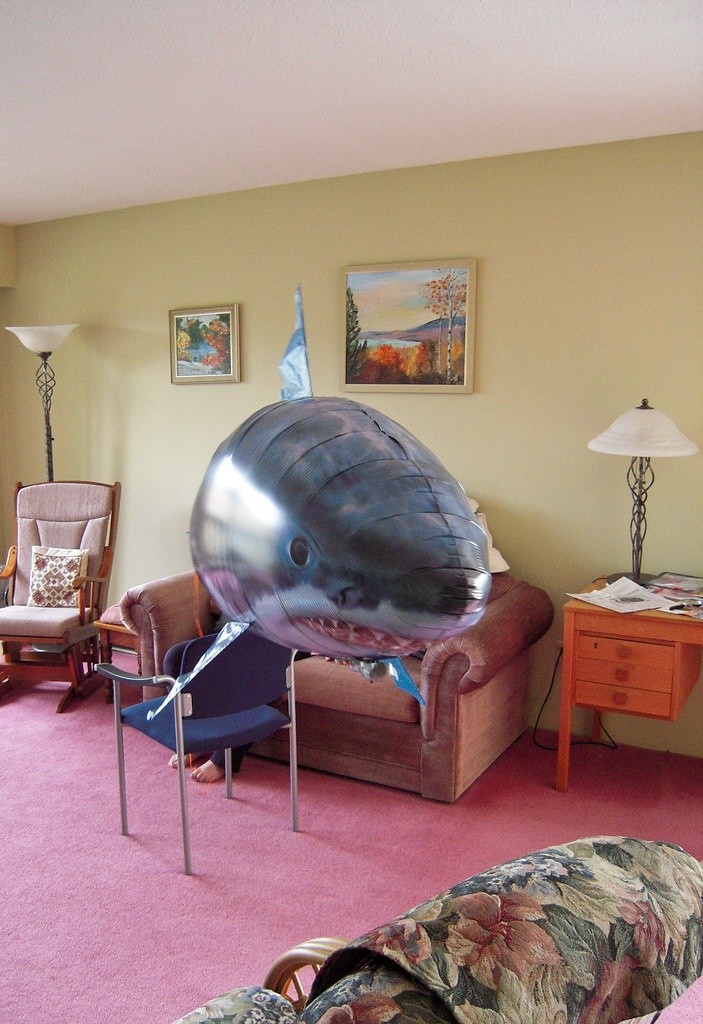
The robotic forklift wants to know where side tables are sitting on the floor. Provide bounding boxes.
[93,620,143,704]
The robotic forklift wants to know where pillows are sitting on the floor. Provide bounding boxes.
[26,545,90,608]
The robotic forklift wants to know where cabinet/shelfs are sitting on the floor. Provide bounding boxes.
[556,575,703,793]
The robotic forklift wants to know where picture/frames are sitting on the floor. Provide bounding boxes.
[339,258,476,394]
[168,304,241,385]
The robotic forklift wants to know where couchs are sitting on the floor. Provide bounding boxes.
[120,566,554,802]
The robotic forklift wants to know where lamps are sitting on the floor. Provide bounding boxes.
[587,398,699,587]
[4,323,79,481]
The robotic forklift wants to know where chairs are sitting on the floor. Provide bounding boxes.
[0,481,121,713]
[165,836,703,1024]
[95,633,301,875]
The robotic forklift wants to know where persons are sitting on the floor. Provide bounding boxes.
[166,596,351,783]
